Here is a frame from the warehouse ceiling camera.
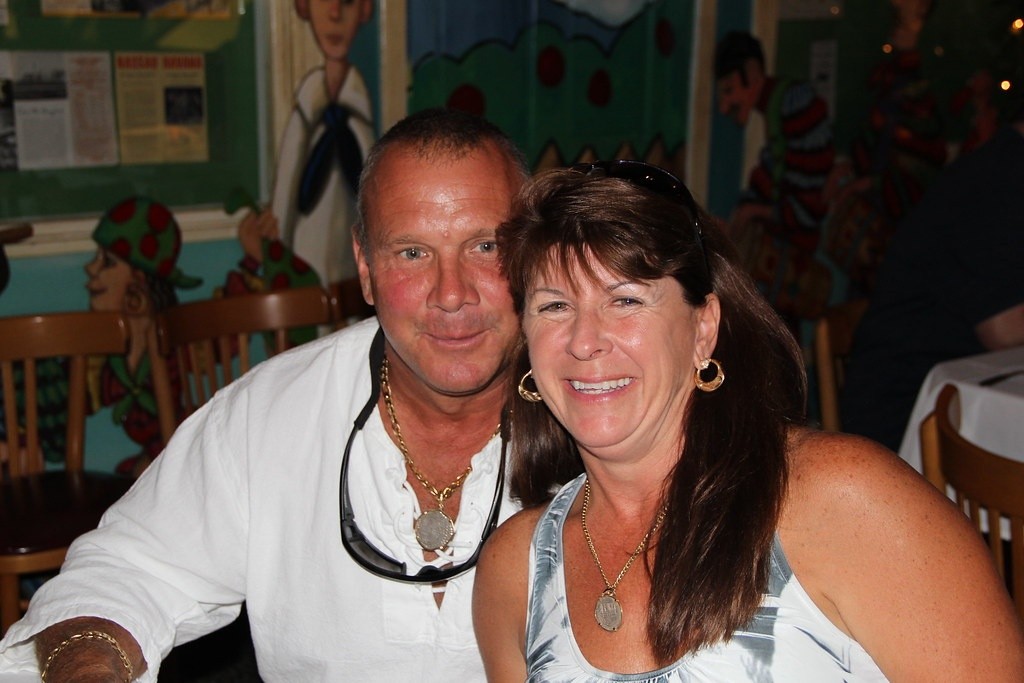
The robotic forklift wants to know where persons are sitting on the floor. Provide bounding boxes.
[836,93,1023,455]
[0,105,528,683]
[470,150,1024,683]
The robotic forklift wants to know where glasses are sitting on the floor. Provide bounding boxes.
[568,160,711,278]
[339,425,507,584]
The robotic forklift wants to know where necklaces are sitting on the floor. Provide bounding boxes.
[581,478,669,632]
[380,352,515,553]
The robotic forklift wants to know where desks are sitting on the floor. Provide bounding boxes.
[910,345,1024,450]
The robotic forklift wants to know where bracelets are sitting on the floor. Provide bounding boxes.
[40,630,134,683]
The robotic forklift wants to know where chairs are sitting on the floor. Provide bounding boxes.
[0,310,133,638]
[916,383,1024,623]
[147,280,378,449]
